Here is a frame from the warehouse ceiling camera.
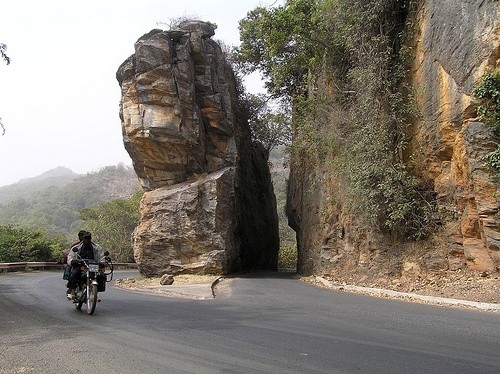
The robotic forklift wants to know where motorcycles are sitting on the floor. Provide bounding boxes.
[64,247,112,315]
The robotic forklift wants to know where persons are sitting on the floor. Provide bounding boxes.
[67,231,106,302]
[65,229,88,296]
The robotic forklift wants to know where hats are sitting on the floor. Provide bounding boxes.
[78,230,87,236]
[82,232,91,238]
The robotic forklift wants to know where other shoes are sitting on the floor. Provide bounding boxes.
[97,293,102,302]
[67,290,74,299]
